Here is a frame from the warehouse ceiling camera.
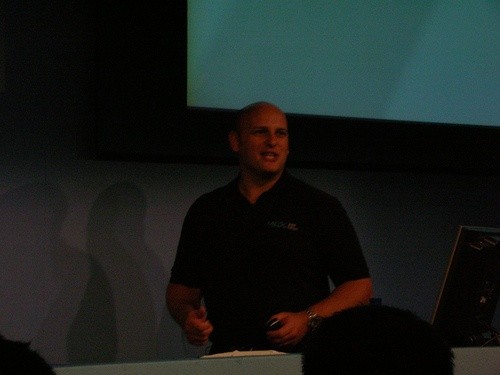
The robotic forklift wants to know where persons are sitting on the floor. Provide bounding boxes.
[166,102,374,354]
[300,303,456,375]
[0,337,56,375]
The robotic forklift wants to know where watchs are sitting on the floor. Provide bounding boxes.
[304,308,323,332]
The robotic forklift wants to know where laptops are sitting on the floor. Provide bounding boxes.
[430,226,500,346]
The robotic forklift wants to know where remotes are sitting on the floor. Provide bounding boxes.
[268,319,282,330]
